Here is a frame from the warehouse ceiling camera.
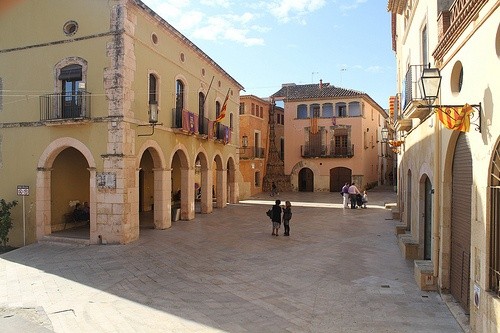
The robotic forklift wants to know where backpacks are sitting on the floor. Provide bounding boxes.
[266,207,273,219]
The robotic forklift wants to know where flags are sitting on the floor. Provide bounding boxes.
[392,149,400,154]
[216,92,231,120]
[389,141,403,146]
[434,103,473,132]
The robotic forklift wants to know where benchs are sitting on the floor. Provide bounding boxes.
[64,213,89,231]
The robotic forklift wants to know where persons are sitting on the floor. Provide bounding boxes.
[282,200,292,237]
[362,190,368,208]
[342,182,351,209]
[348,182,360,209]
[271,200,282,236]
[74,202,90,228]
[271,181,279,196]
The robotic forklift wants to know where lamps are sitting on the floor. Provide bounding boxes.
[416,62,481,132]
[138,98,161,136]
[236,134,248,154]
[380,125,403,150]
